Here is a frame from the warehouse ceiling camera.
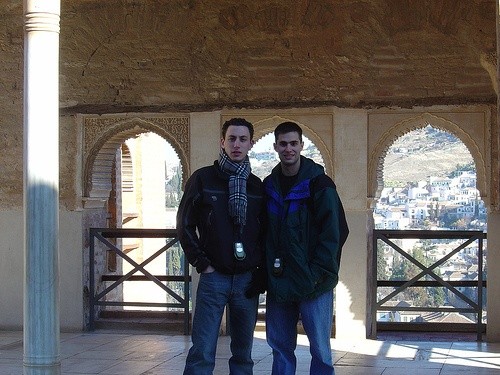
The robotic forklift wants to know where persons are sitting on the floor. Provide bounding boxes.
[263,122,350,375]
[175,118,267,375]
